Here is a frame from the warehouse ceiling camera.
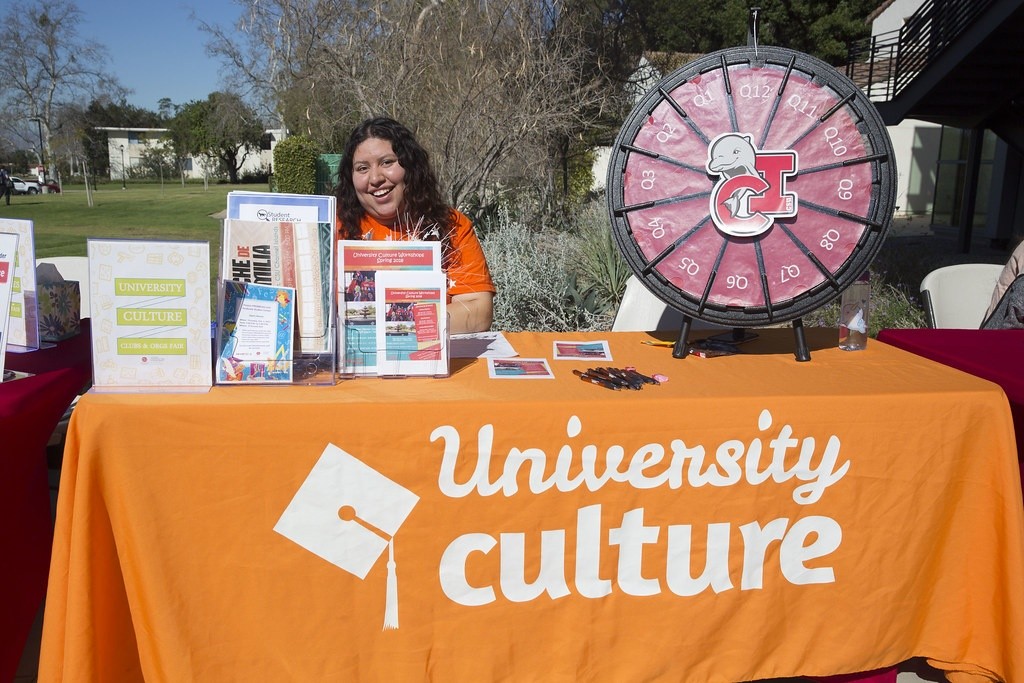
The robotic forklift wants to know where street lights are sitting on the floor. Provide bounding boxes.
[89,143,97,190]
[28,118,47,186]
[120,144,128,190]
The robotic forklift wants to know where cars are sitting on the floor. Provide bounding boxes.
[8,176,39,195]
[25,179,60,194]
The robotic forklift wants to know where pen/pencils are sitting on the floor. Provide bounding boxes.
[570,367,661,393]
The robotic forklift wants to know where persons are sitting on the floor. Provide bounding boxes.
[2,170,14,205]
[333,117,497,337]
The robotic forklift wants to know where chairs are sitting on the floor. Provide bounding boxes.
[915,260,1010,327]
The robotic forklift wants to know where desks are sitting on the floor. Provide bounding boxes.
[4,326,1024,682]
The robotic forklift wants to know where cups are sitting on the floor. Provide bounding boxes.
[838,280,871,350]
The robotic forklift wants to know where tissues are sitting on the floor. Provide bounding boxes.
[33,258,85,347]
